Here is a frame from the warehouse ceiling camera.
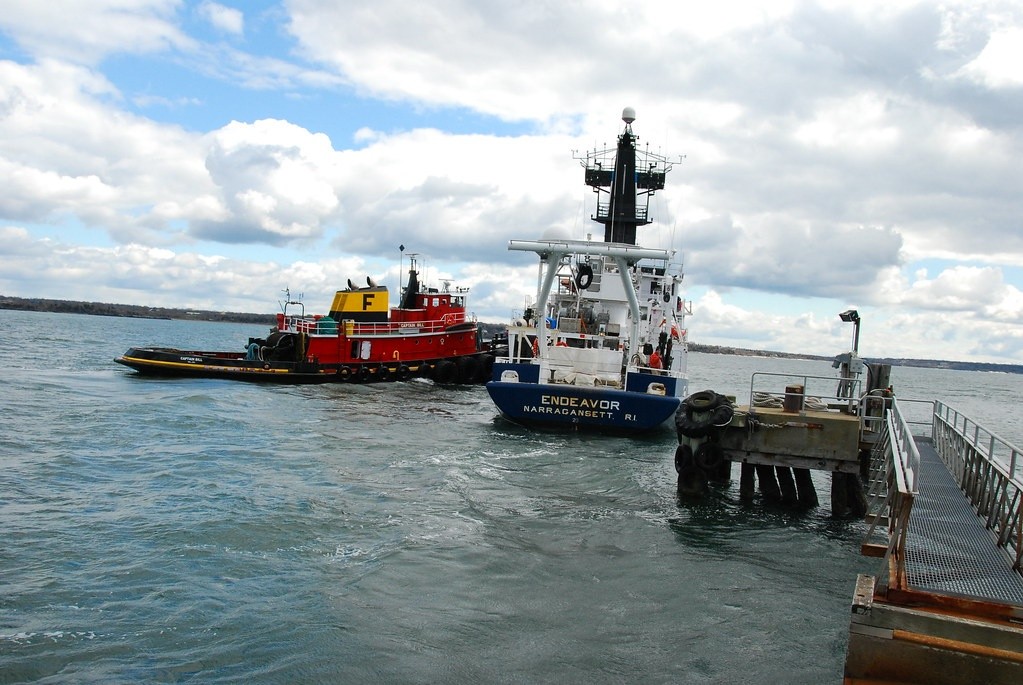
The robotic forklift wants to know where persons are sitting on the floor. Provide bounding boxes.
[649,346,661,369]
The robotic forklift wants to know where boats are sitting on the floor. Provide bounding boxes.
[114,244,535,387]
[487,107,693,434]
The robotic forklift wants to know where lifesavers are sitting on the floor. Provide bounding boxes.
[376,366,390,381]
[418,354,495,385]
[695,441,725,473]
[533,340,537,356]
[337,366,352,381]
[397,364,410,379]
[674,390,734,440]
[556,342,567,347]
[356,367,370,381]
[675,445,692,474]
[446,315,454,325]
[496,348,509,357]
